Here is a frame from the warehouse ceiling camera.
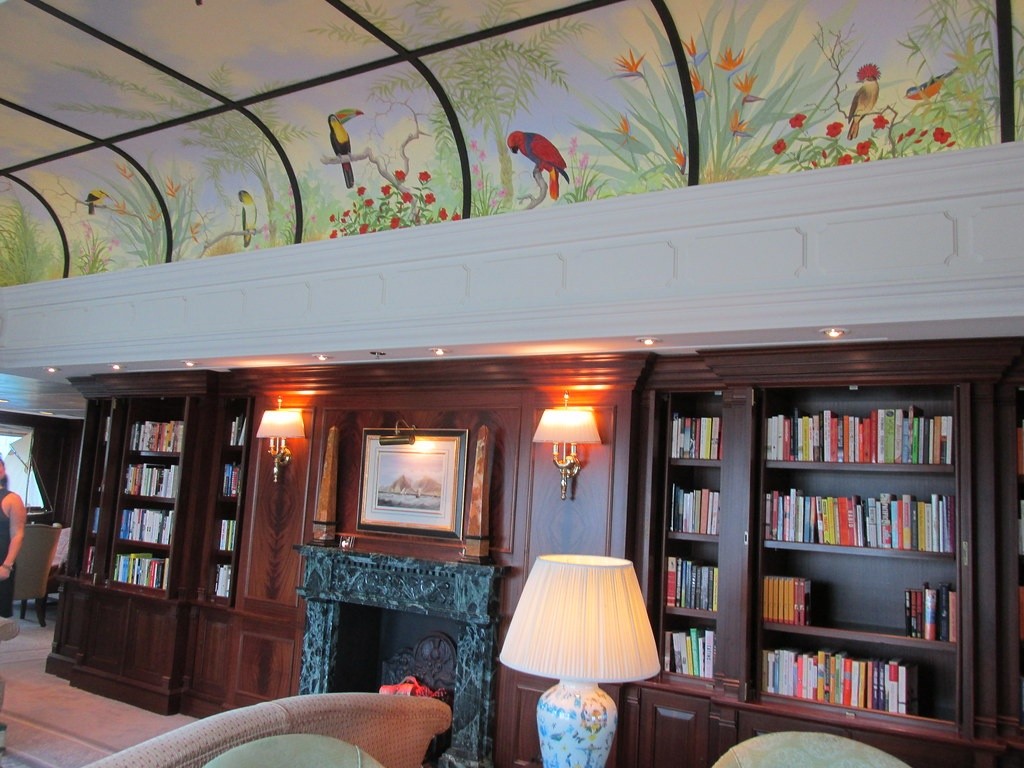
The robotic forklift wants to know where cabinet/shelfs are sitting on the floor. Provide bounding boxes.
[643,378,1024,730]
[60,393,253,611]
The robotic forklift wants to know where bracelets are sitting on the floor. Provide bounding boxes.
[2,564,13,570]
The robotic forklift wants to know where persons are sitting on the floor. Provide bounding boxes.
[0,460,27,619]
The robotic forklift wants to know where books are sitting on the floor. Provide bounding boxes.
[672,413,722,460]
[765,489,955,553]
[762,647,918,715]
[87,507,101,573]
[215,414,246,598]
[667,557,717,612]
[120,508,174,544]
[665,628,716,677]
[115,553,169,590]
[905,582,956,642]
[768,403,952,464]
[130,420,184,453]
[671,484,718,535]
[127,462,177,498]
[764,574,812,625]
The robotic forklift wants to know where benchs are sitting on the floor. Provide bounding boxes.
[78,693,453,768]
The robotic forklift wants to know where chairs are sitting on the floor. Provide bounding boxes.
[204,733,385,768]
[711,731,913,768]
[13,523,63,628]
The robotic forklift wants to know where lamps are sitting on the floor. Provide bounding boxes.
[498,554,661,768]
[378,417,417,445]
[256,395,306,483]
[532,389,602,500]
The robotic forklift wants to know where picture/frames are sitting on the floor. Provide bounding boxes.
[356,427,469,541]
